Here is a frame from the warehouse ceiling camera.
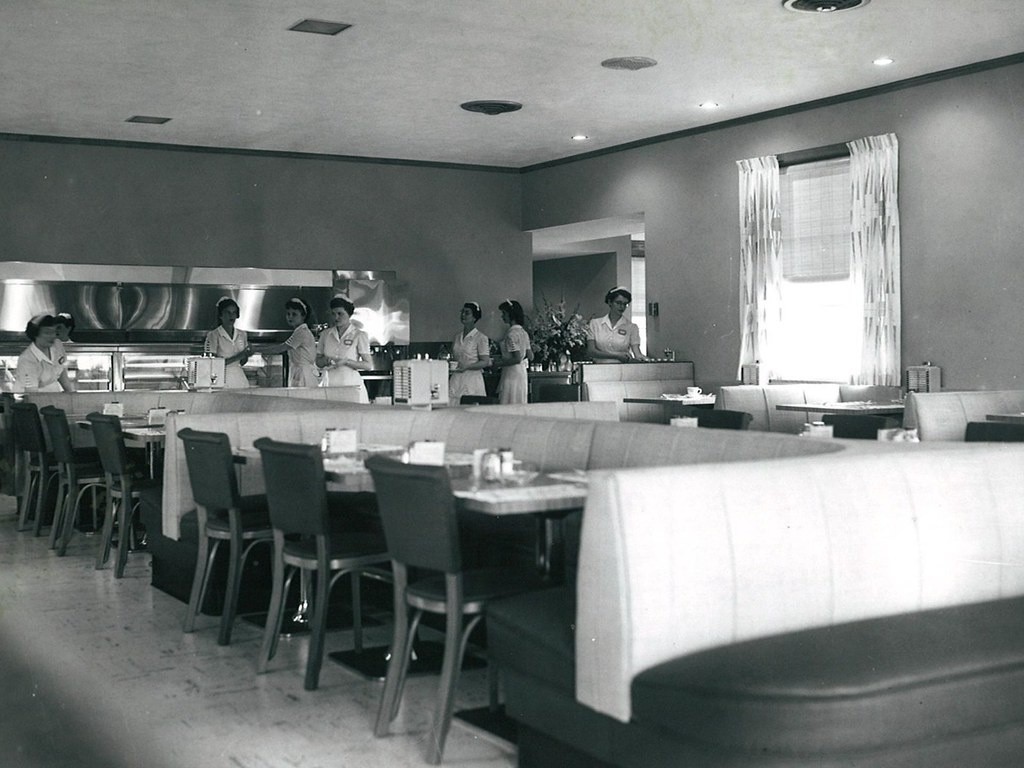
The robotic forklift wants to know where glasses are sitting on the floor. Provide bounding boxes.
[612,298,630,308]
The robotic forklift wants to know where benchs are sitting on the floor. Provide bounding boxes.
[713,384,842,437]
[580,379,695,423]
[28,386,1024,768]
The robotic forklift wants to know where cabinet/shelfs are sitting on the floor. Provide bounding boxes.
[365,375,573,404]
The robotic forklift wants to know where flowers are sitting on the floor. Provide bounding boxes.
[523,285,595,372]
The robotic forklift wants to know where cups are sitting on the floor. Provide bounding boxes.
[687,387,702,395]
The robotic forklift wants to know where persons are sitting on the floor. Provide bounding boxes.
[316,294,373,404]
[450,302,489,399]
[490,301,535,406]
[14,315,75,393]
[250,297,318,388]
[205,297,252,387]
[588,287,649,363]
[55,313,75,343]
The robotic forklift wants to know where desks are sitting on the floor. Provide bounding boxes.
[237,441,403,485]
[448,472,586,589]
[75,417,165,480]
[776,403,905,416]
[623,398,716,425]
[985,414,1023,423]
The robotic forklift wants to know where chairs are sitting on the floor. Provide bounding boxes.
[541,384,582,402]
[822,414,900,441]
[691,410,753,431]
[965,422,1024,443]
[2,391,551,766]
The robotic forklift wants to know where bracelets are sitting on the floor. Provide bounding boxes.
[345,359,350,366]
